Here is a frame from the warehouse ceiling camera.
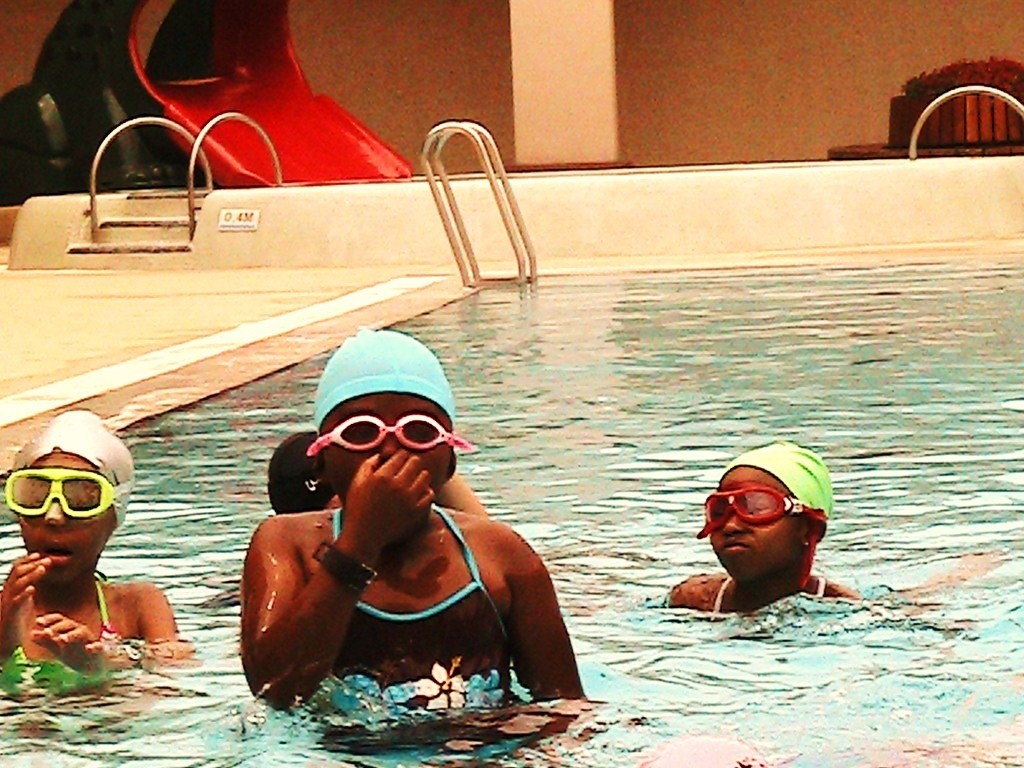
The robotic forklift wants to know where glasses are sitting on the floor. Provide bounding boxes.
[305,413,472,453]
[695,485,827,541]
[4,467,115,519]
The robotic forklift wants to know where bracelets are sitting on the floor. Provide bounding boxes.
[312,541,378,593]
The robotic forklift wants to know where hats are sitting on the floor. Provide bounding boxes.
[717,439,835,514]
[10,410,136,526]
[306,329,459,424]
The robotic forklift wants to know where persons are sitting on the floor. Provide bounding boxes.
[240,331,587,704]
[267,431,490,518]
[0,407,179,675]
[667,440,864,614]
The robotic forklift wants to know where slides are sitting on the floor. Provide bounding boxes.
[78,0,413,190]
